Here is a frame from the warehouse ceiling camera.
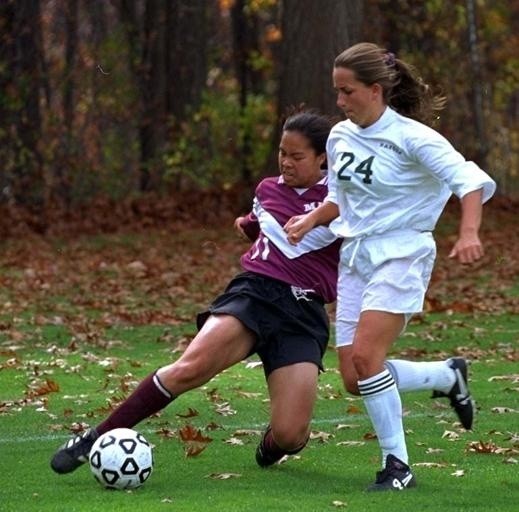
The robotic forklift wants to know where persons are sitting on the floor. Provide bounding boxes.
[49,107,348,476]
[283,39,496,495]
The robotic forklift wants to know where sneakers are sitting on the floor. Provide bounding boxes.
[255,424,286,467]
[428,357,474,430]
[367,454,416,491]
[50,427,97,474]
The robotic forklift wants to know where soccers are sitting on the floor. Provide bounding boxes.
[89,428,154,492]
